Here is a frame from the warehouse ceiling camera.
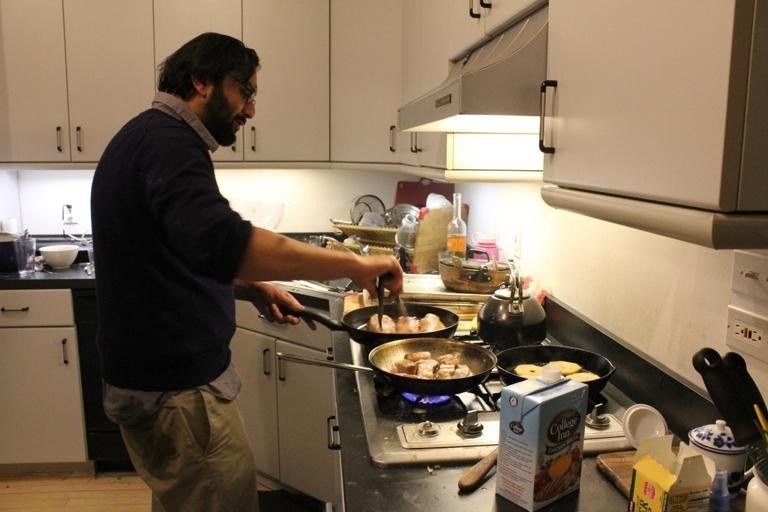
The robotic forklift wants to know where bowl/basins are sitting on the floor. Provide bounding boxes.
[38,244,79,270]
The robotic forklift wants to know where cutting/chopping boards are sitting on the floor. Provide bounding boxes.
[595,446,746,511]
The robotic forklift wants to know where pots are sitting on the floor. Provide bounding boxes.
[494,345,615,397]
[438,250,512,294]
[275,338,498,395]
[275,303,459,348]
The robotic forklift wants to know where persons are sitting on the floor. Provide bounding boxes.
[88,31,406,510]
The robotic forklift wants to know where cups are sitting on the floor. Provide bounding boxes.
[13,238,36,275]
[687,419,755,499]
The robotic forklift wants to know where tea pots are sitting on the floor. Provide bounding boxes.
[476,263,548,348]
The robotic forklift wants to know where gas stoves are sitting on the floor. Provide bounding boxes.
[347,330,635,468]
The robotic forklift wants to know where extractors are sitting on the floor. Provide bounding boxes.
[398,2,550,133]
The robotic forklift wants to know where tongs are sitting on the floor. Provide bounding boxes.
[377,275,408,331]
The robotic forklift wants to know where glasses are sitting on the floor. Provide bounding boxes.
[226,71,257,105]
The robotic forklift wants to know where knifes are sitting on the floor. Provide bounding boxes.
[690,348,768,484]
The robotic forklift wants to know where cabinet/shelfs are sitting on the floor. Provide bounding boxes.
[221,294,328,504]
[395,1,543,186]
[1,287,89,463]
[153,0,331,170]
[1,1,153,169]
[539,1,767,255]
[328,0,395,170]
[445,1,548,63]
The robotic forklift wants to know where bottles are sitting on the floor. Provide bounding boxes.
[709,468,730,512]
[446,192,467,259]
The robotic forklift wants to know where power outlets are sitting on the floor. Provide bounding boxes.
[725,305,767,364]
[62,204,79,225]
